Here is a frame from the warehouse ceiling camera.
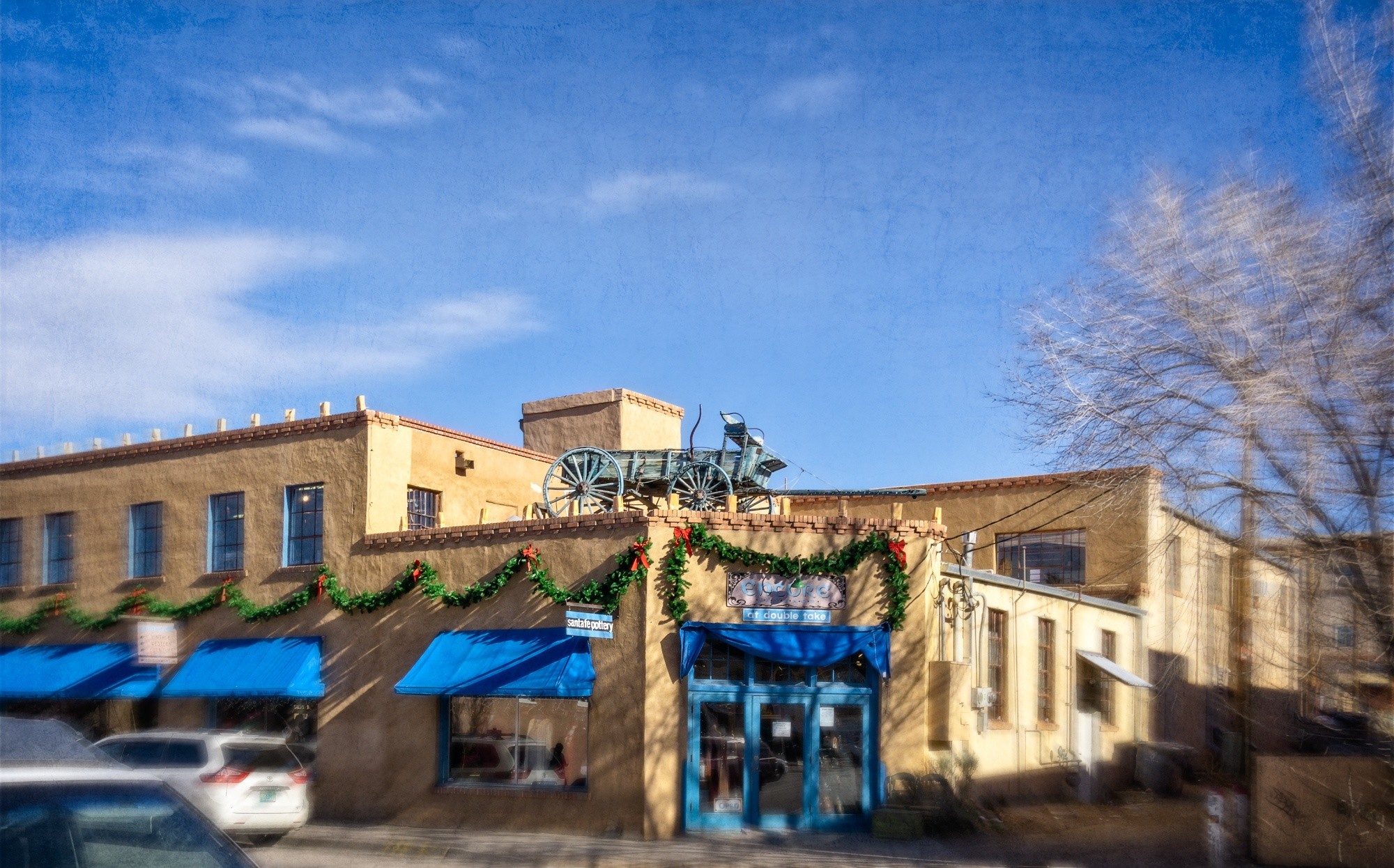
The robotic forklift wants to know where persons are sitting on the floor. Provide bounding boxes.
[545,739,569,786]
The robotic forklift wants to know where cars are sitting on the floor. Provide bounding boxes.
[0,718,260,868]
[700,736,784,793]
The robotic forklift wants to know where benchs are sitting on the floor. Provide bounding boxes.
[718,410,765,450]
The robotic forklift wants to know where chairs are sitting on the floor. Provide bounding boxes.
[0,801,88,867]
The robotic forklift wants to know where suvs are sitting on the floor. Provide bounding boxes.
[88,728,309,847]
[449,734,567,789]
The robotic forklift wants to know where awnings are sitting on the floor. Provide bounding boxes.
[394,625,596,699]
[0,638,157,701]
[160,636,324,699]
